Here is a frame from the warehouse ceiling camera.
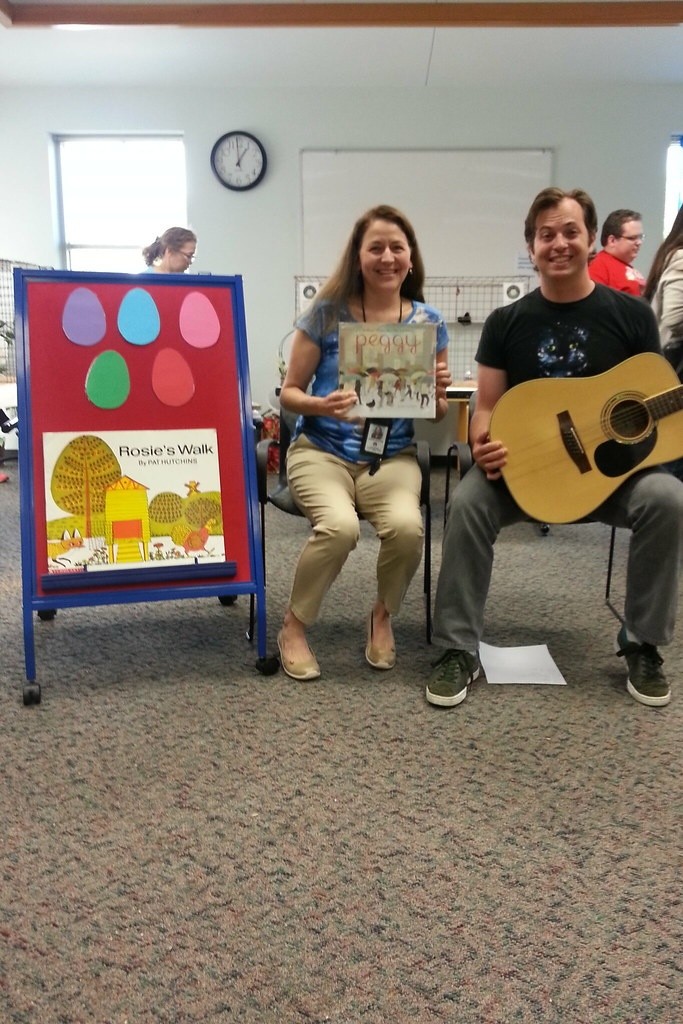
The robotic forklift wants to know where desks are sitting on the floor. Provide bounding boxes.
[274,386,479,472]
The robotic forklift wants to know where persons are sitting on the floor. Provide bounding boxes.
[142,227,197,273]
[644,204,683,384]
[588,209,646,296]
[372,426,382,439]
[427,188,683,705]
[277,206,453,679]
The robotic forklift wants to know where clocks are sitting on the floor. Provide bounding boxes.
[209,131,268,192]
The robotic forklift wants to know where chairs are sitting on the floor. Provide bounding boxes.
[255,406,432,645]
[444,390,628,626]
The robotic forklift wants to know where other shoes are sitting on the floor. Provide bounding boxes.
[276,629,322,681]
[364,607,397,670]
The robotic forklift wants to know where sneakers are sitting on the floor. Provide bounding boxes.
[424,647,482,708]
[612,621,671,707]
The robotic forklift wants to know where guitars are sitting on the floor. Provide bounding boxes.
[488,352,682,525]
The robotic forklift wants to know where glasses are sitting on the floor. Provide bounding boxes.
[613,232,646,240]
[172,248,196,263]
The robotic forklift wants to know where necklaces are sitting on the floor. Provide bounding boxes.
[361,295,404,325]
